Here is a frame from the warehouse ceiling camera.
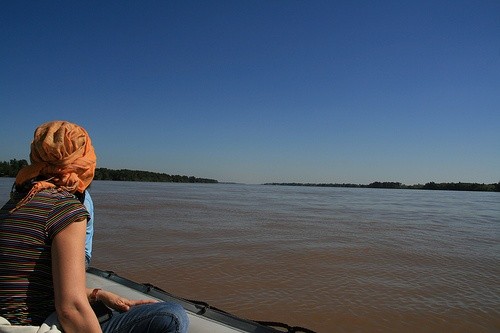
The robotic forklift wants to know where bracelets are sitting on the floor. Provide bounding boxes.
[90,289,101,301]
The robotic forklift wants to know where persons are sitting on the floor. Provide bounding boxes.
[0,119,189,333]
[84,189,93,270]
